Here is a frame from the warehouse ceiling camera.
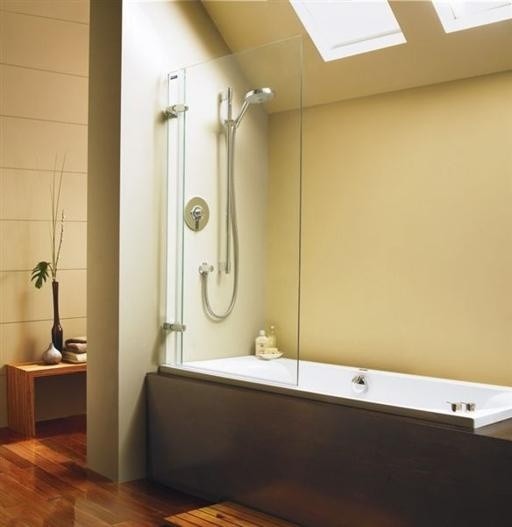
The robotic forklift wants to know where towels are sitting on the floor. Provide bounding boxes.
[63,336,87,354]
[62,351,87,363]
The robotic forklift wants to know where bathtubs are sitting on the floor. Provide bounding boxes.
[158,356,511,428]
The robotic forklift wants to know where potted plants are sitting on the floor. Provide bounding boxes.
[30,150,72,353]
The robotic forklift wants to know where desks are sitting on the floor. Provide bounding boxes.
[5,360,87,437]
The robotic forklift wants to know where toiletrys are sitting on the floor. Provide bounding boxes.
[254,329,267,355]
[267,324,276,349]
